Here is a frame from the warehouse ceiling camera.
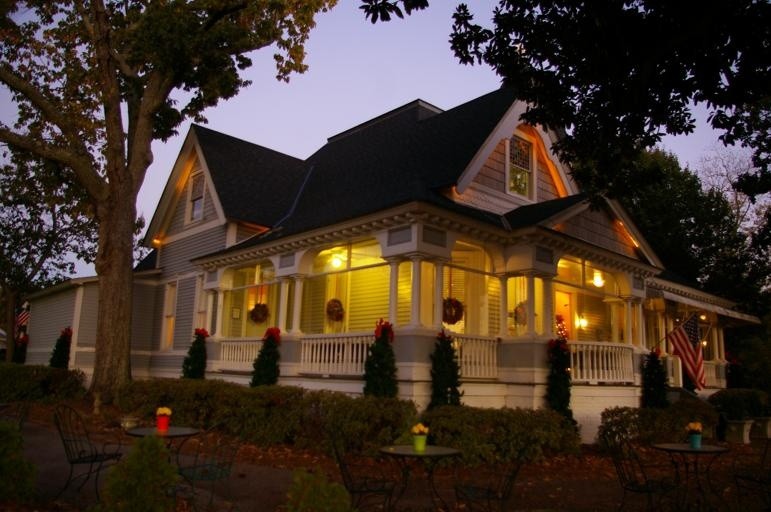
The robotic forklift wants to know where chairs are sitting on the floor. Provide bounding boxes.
[327,428,397,512]
[734,440,771,512]
[176,414,244,512]
[54,405,124,504]
[453,462,526,512]
[599,430,676,512]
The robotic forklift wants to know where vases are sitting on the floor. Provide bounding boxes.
[413,434,427,452]
[691,435,701,449]
[156,416,169,433]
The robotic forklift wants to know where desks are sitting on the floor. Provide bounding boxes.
[123,428,200,510]
[650,443,732,512]
[379,445,465,512]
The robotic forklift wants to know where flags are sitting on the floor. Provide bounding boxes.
[668,311,706,392]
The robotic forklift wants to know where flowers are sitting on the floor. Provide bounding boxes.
[685,422,702,434]
[155,406,173,417]
[410,423,429,434]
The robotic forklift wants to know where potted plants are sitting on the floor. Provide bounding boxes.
[707,388,770,444]
